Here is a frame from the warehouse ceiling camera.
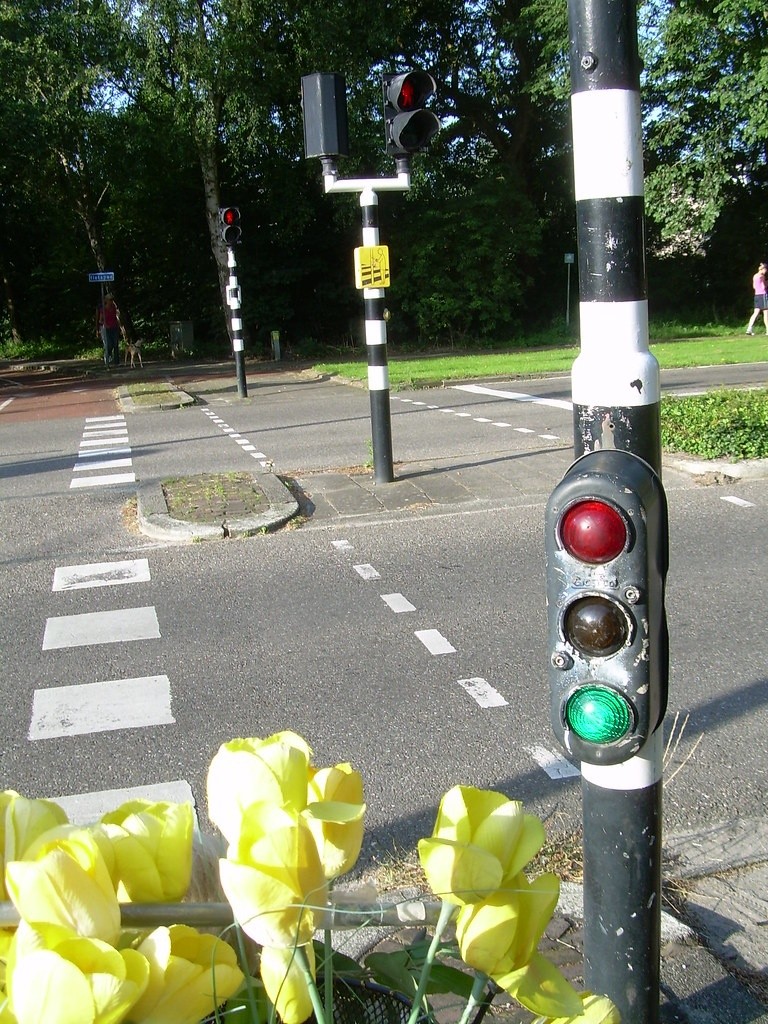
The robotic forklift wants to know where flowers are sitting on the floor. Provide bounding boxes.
[0,731,621,1023]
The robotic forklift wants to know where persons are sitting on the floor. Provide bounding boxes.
[745,262,768,337]
[96,294,124,372]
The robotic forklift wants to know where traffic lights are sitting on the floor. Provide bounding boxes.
[542,449,670,765]
[384,68,442,154]
[219,207,243,246]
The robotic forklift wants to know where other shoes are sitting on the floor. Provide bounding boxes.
[104,364,109,370]
[115,364,124,368]
[745,331,755,336]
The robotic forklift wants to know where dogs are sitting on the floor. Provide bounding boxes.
[124,337,147,369]
